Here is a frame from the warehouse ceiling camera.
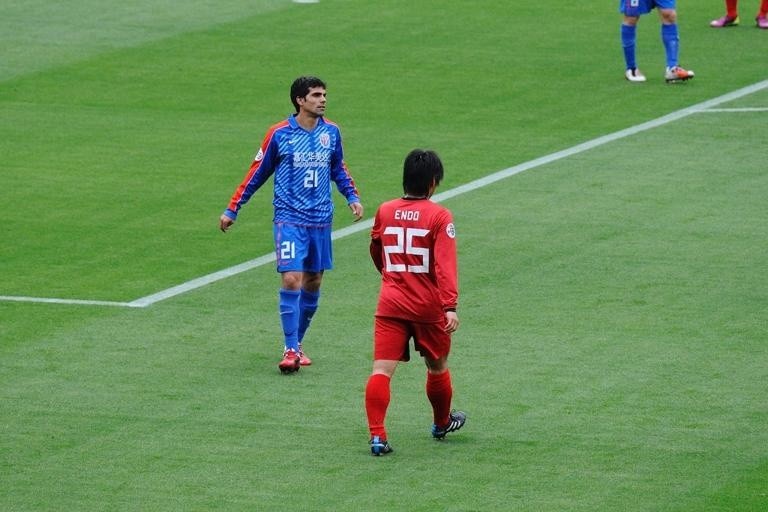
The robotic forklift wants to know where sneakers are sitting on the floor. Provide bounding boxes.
[367,435,393,457]
[623,68,647,83]
[278,346,300,373]
[431,409,468,439]
[297,344,312,366]
[663,66,695,83]
[754,15,768,30]
[709,14,740,28]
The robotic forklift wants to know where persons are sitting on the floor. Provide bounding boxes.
[618,1,694,85]
[220,75,365,374]
[364,148,468,458]
[709,1,768,29]
